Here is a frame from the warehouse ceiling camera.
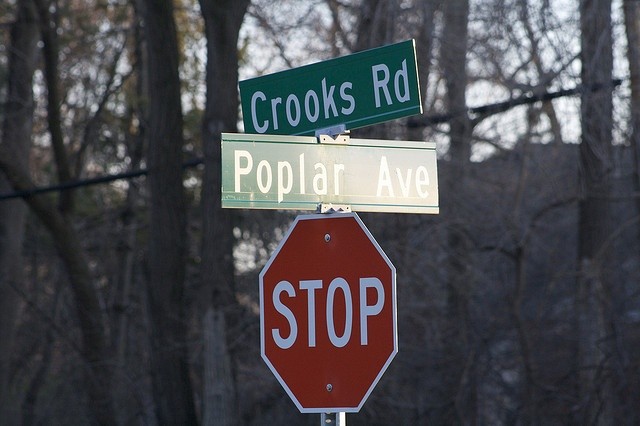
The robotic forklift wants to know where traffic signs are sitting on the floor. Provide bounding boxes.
[220,133,439,214]
[237,37,423,134]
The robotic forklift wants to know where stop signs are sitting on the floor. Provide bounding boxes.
[260,213,398,413]
